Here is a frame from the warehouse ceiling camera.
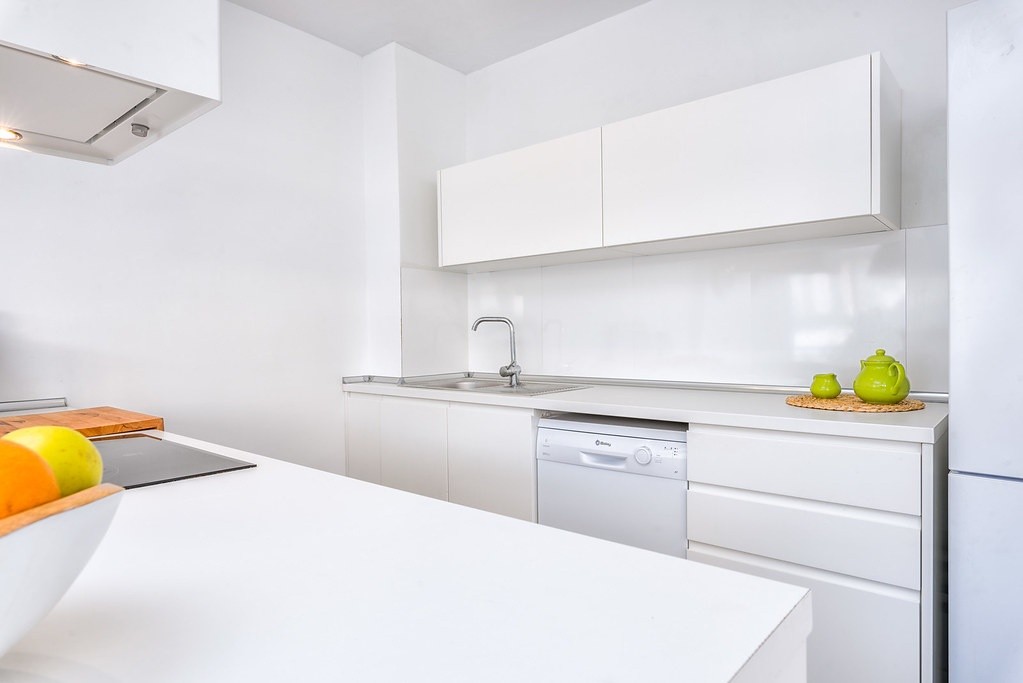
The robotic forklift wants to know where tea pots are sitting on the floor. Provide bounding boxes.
[853,349,910,404]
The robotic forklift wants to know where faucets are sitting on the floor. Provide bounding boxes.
[471,317,522,387]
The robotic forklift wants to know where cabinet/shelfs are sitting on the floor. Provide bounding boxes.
[340,392,947,683]
[437,51,902,272]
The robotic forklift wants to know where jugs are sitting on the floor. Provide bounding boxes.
[811,373,841,398]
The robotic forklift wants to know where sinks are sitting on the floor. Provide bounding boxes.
[397,377,510,393]
